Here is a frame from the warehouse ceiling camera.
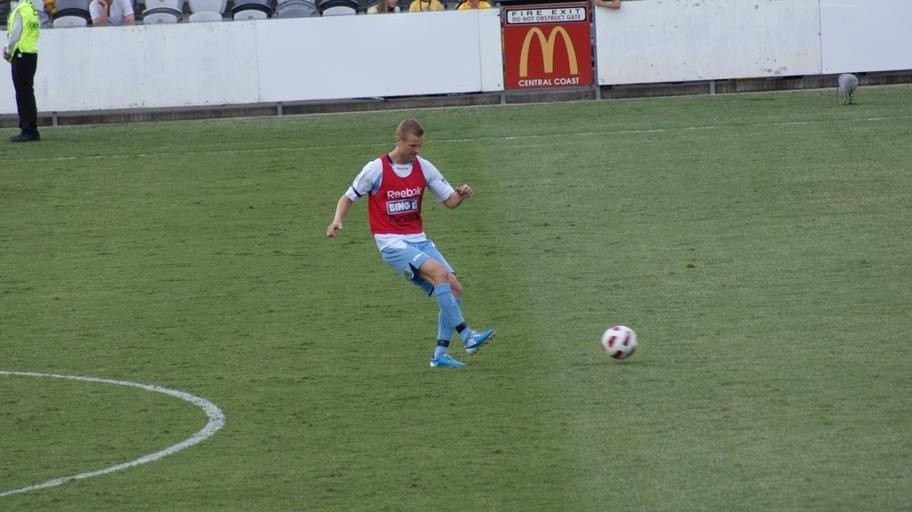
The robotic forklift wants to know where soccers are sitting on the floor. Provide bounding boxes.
[602,325,636,358]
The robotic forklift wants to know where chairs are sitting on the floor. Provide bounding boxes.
[40,0,399,28]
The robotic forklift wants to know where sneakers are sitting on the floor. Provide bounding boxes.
[462,328,496,356]
[9,133,41,142]
[430,353,465,367]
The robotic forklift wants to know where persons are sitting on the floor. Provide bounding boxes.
[326,117,495,368]
[590,0,622,11]
[89,1,135,25]
[367,1,400,14]
[408,0,444,14]
[458,1,492,10]
[2,0,42,143]
